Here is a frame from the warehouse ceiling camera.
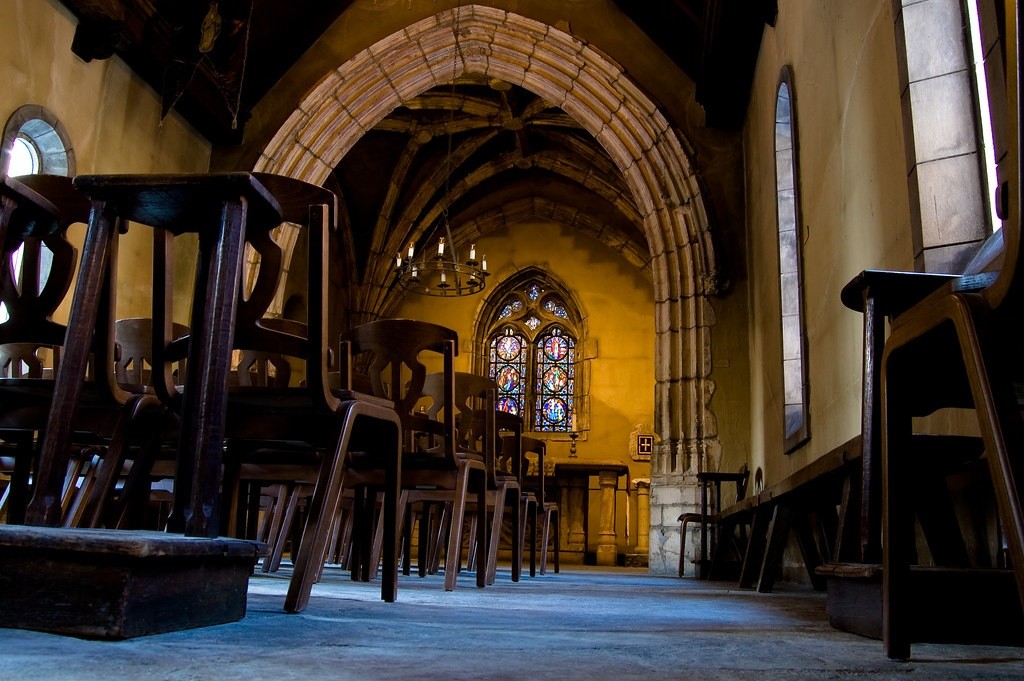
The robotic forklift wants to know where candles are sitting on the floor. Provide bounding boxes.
[572,408,577,431]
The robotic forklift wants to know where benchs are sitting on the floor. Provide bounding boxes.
[712,435,881,597]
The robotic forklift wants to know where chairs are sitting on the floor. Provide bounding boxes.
[878,0,1024,661]
[0,170,561,615]
[677,463,751,578]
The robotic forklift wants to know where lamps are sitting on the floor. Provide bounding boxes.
[393,0,491,297]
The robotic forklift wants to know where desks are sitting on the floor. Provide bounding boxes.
[498,457,631,566]
